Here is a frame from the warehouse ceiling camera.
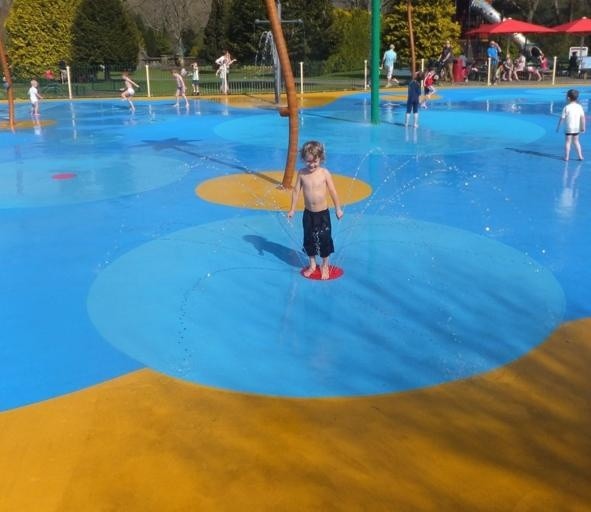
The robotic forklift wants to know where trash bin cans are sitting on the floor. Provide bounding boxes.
[453,59,465,82]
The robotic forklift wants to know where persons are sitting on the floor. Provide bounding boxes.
[28,112,42,139]
[216,50,237,93]
[60,57,68,82]
[173,106,191,118]
[380,43,398,88]
[568,51,578,78]
[122,108,140,128]
[194,95,201,117]
[427,37,549,84]
[170,68,190,108]
[403,124,420,155]
[119,71,141,110]
[391,70,429,128]
[280,280,346,385]
[286,140,345,282]
[43,66,57,86]
[26,79,44,117]
[552,160,585,230]
[556,89,587,162]
[190,62,201,97]
[423,65,439,110]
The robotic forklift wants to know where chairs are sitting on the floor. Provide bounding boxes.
[430,52,558,81]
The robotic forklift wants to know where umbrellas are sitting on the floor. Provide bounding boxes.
[545,15,591,56]
[466,16,557,58]
